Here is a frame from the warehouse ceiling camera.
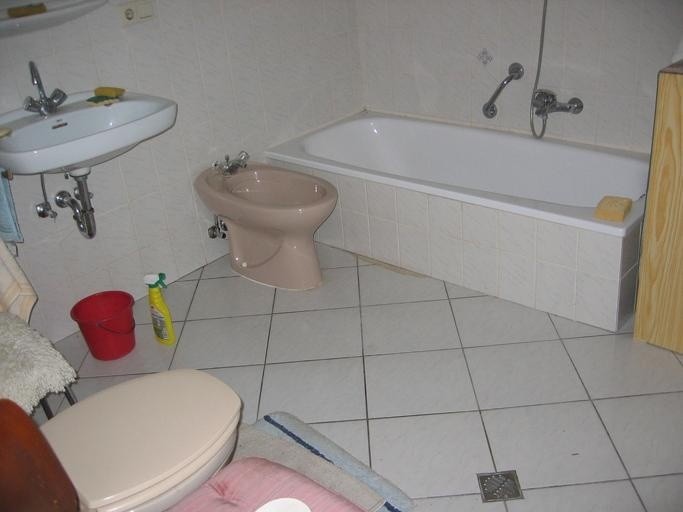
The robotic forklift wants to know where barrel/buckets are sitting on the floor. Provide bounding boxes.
[70,291,136,359]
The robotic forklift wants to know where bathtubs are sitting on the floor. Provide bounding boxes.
[269,111,652,237]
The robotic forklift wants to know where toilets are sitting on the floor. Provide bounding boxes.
[0,369,242,512]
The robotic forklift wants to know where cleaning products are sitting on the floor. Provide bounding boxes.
[143,273,175,345]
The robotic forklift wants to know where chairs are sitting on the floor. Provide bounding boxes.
[164,456,363,512]
[0,311,83,419]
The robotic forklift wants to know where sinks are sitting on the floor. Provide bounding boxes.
[0,91,176,175]
[194,160,338,290]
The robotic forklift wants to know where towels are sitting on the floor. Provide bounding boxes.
[0,237,39,322]
[0,166,24,243]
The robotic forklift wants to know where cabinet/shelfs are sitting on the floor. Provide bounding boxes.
[630,73,683,353]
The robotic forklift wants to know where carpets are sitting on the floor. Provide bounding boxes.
[227,410,415,512]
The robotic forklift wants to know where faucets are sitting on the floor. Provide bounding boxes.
[29,61,48,104]
[226,159,249,173]
